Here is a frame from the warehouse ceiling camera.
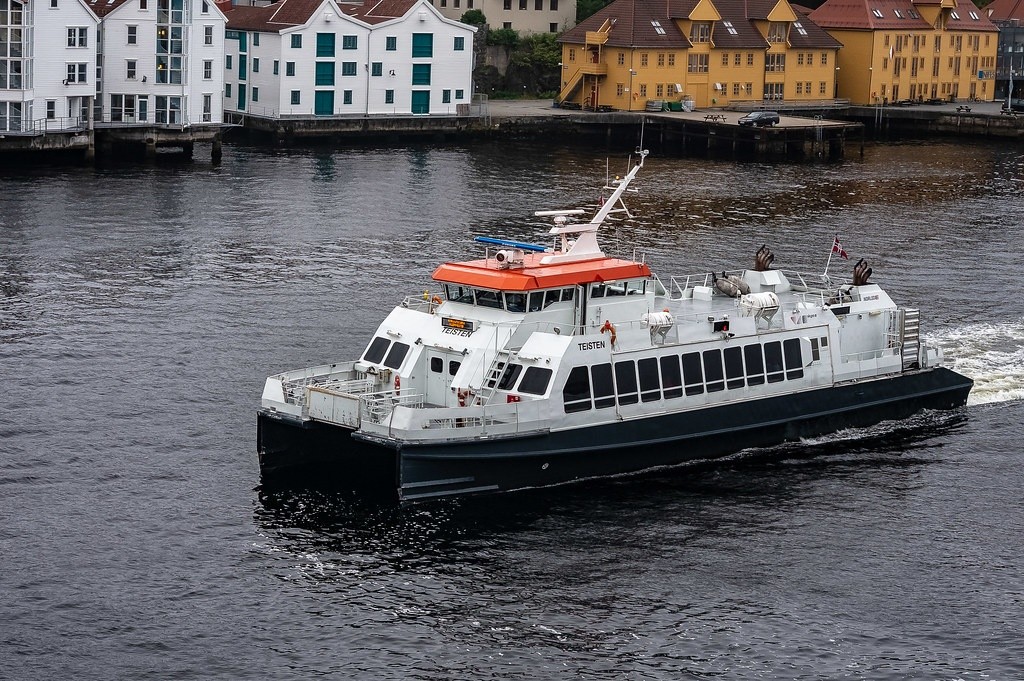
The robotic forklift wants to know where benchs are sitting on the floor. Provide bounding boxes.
[703,116,727,119]
[560,104,582,108]
[596,107,614,110]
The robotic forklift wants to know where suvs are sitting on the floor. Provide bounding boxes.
[737,110,780,128]
[1002,97,1024,112]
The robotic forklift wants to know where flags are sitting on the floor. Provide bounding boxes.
[833,238,847,259]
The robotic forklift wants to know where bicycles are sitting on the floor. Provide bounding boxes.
[967,94,982,104]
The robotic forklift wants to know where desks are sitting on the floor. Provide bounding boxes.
[564,102,580,110]
[704,114,726,122]
[598,105,613,112]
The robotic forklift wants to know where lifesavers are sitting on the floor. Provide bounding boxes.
[599,325,616,345]
[458,390,481,407]
[394,374,400,395]
[429,295,441,312]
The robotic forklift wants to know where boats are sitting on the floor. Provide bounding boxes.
[256,121,975,510]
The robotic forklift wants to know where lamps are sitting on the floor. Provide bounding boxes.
[141,76,147,82]
[63,79,69,85]
[389,70,396,76]
